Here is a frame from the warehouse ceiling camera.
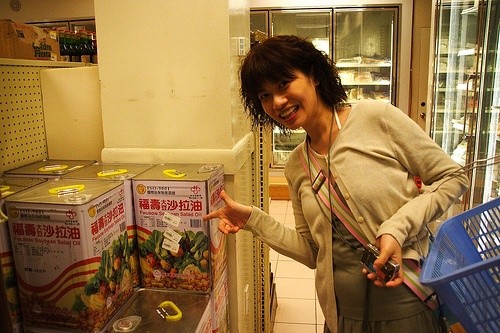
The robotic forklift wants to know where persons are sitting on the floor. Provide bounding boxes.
[203,35,470,333]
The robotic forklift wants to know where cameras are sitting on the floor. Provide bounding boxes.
[359,244,400,285]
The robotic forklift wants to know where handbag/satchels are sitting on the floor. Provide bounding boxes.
[434,304,468,333]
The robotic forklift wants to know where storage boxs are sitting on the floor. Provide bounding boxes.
[0,19,61,60]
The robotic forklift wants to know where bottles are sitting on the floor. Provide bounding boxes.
[75,30,98,64]
[250,30,266,49]
[59,30,75,62]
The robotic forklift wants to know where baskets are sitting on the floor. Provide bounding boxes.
[421,154,500,333]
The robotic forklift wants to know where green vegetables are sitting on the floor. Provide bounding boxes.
[138,229,208,273]
[71,229,138,309]
